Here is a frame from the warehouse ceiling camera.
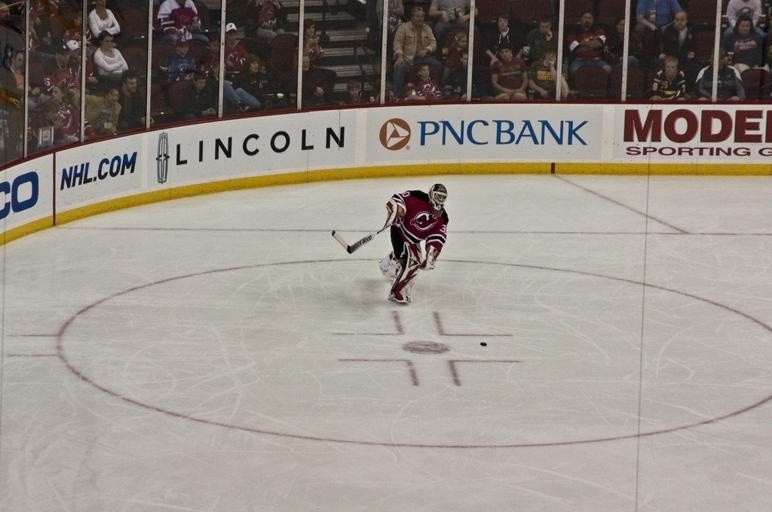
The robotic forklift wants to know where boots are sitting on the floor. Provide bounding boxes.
[388,289,408,303]
[379,254,395,280]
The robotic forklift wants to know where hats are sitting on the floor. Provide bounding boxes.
[225,23,238,32]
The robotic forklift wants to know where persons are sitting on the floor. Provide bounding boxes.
[375,183,449,304]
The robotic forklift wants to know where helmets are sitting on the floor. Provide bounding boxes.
[428,184,448,198]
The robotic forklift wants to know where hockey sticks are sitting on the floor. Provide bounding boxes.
[332,224,391,254]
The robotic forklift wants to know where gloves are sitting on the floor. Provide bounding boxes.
[420,246,440,272]
